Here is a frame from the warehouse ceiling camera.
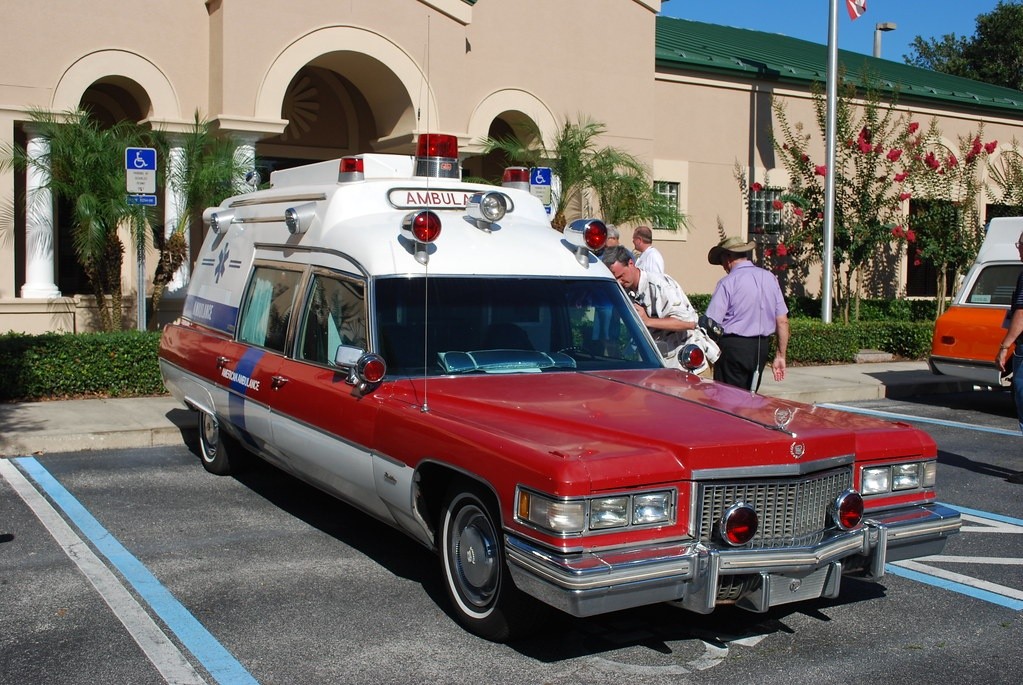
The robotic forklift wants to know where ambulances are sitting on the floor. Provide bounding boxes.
[158,132,965,644]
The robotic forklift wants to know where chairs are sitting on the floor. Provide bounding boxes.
[483,321,536,352]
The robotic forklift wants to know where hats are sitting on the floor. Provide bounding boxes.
[708,237,756,266]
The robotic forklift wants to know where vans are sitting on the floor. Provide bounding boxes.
[928,217,1023,396]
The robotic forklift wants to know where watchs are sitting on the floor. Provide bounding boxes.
[1000,344,1010,350]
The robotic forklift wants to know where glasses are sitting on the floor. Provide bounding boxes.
[1015,242,1023,248]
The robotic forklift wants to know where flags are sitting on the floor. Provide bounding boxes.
[846,0,867,21]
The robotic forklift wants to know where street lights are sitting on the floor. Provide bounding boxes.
[872,22,896,59]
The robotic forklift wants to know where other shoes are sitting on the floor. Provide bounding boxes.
[1008,472,1023,484]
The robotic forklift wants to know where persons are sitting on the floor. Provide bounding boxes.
[705,236,789,393]
[592,224,721,380]
[995,232,1023,484]
